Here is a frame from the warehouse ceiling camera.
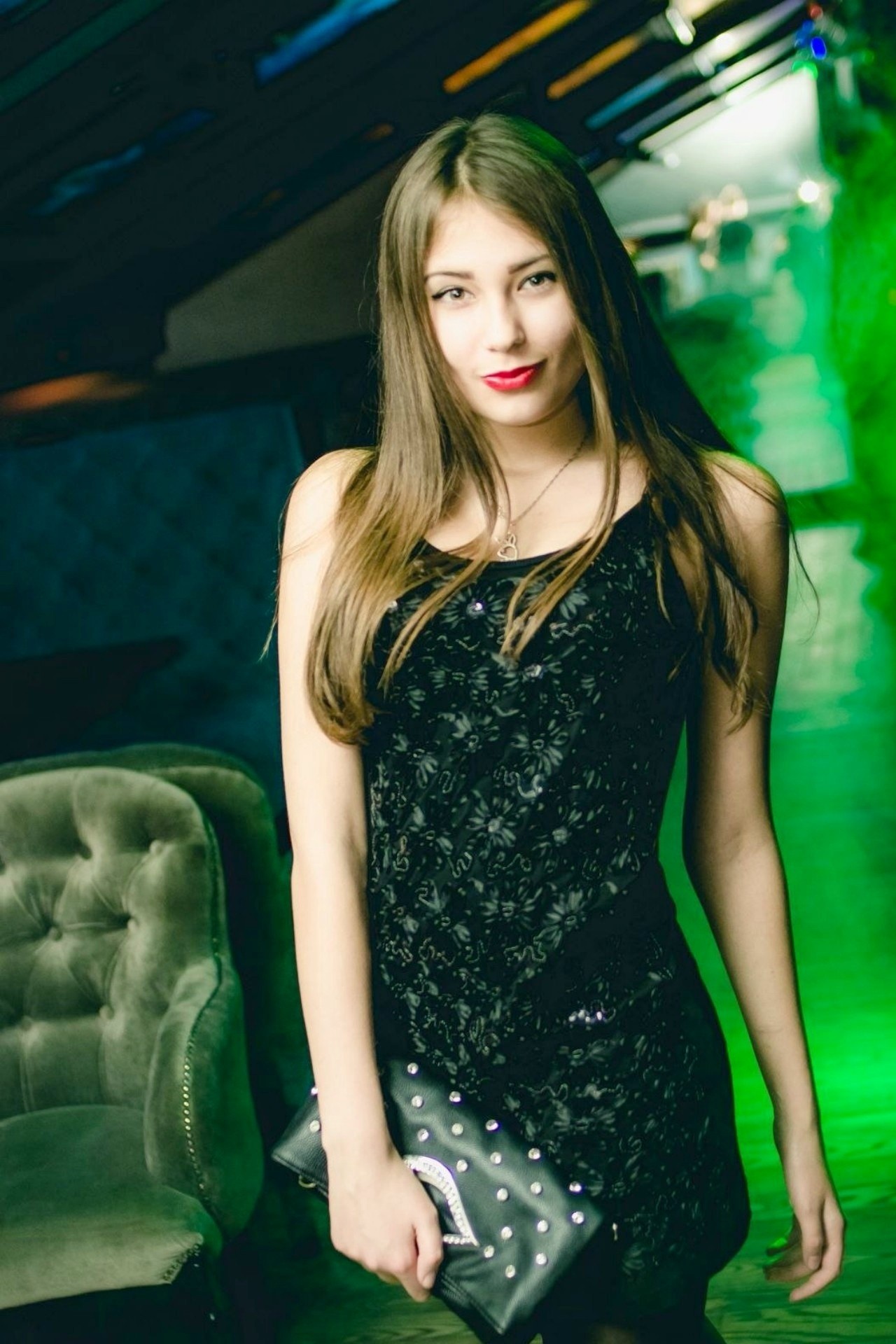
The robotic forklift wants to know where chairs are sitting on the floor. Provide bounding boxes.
[0,735,315,1344]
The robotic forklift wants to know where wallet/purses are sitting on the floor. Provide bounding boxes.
[269,1047,606,1338]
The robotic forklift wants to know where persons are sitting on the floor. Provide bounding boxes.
[279,115,846,1344]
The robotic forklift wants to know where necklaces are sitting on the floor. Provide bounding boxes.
[497,427,590,561]
